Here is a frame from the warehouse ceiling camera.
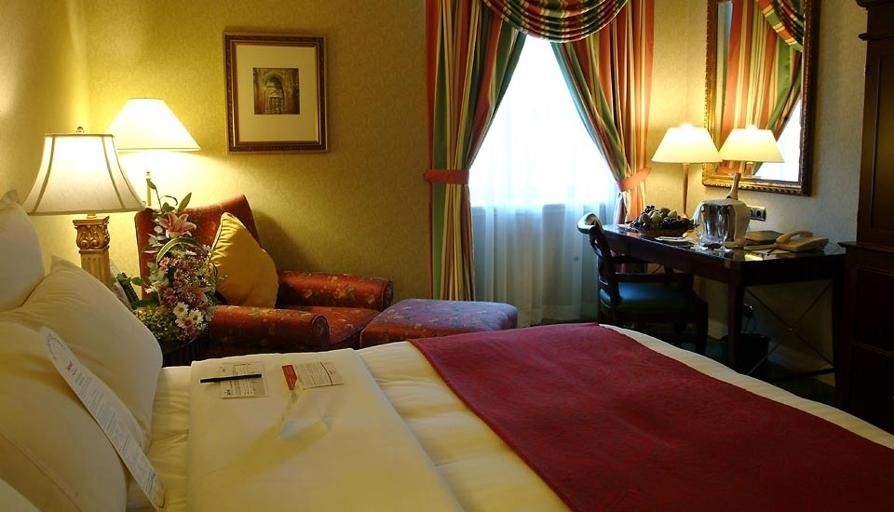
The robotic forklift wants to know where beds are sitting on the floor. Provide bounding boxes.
[0,187,894,511]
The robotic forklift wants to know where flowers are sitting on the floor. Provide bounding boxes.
[118,180,218,342]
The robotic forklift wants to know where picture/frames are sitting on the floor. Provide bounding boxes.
[221,29,331,155]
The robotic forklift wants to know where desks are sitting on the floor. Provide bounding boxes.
[600,221,848,379]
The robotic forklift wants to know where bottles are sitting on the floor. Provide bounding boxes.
[723,171,742,200]
[699,204,733,252]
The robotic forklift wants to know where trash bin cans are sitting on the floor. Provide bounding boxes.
[718,334,771,380]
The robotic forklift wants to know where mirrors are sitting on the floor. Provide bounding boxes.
[701,1,822,197]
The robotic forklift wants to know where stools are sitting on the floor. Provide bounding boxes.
[357,295,518,349]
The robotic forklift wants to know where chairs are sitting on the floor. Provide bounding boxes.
[132,194,394,353]
[575,212,710,355]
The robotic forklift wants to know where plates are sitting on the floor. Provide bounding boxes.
[655,236,694,244]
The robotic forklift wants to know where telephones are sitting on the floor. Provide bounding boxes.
[773,229,829,252]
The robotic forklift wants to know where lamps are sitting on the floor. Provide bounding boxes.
[718,123,786,174]
[23,123,147,289]
[651,123,725,216]
[104,96,204,205]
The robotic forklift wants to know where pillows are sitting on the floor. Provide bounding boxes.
[0,250,167,512]
[200,209,280,313]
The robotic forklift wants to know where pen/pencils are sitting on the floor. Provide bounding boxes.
[767,248,774,253]
[200,374,262,383]
